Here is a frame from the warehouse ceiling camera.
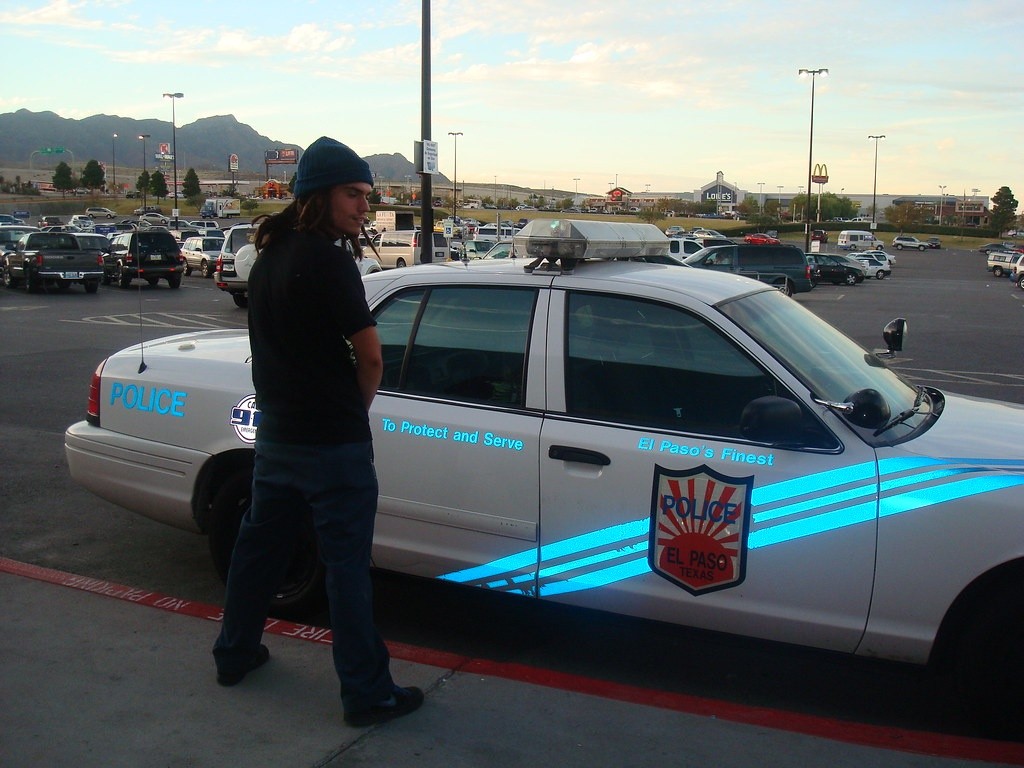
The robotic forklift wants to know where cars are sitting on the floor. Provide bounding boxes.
[369,211,874,259]
[0,190,293,278]
[927,238,942,249]
[978,240,1024,254]
[62,217,1024,745]
[805,250,896,286]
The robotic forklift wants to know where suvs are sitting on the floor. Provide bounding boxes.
[892,235,931,252]
[683,242,814,297]
[100,231,185,288]
[215,222,383,308]
[1009,253,1024,291]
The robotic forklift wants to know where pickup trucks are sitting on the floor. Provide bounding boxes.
[986,252,1021,278]
[1,231,100,292]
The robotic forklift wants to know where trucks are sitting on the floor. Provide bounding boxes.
[199,197,242,220]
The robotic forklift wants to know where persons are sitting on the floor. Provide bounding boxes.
[713,251,730,264]
[212,136,424,727]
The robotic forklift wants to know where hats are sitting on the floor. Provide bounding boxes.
[294,137,373,199]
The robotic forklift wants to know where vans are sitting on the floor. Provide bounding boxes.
[363,229,451,267]
[667,237,706,263]
[838,229,885,251]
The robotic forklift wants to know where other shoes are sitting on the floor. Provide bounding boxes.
[215,643,271,686]
[343,686,424,727]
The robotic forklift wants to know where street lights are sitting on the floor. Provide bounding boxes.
[868,135,886,236]
[797,68,829,253]
[939,186,947,226]
[110,93,184,230]
[448,131,470,223]
